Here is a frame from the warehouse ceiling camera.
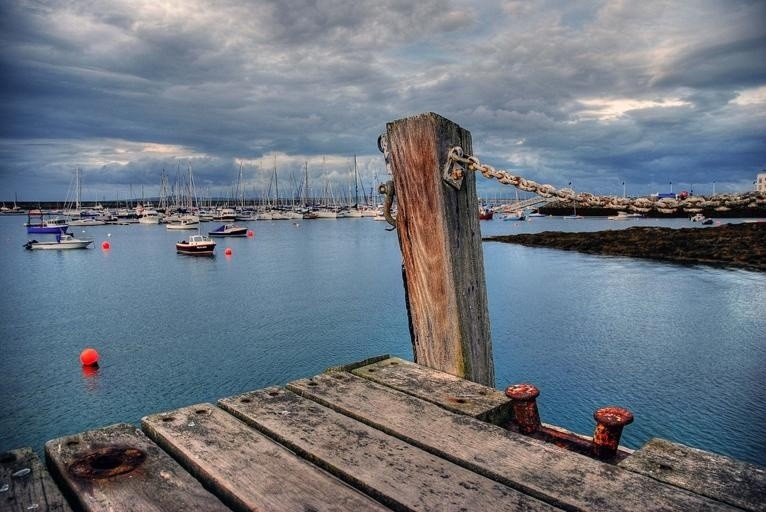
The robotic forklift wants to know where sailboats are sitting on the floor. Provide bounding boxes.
[476,188,545,223]
[0,153,398,237]
[565,199,583,219]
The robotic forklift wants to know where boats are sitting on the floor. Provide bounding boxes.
[608,211,640,221]
[691,216,713,224]
[175,235,218,255]
[22,234,96,250]
[208,222,254,237]
[23,207,71,234]
